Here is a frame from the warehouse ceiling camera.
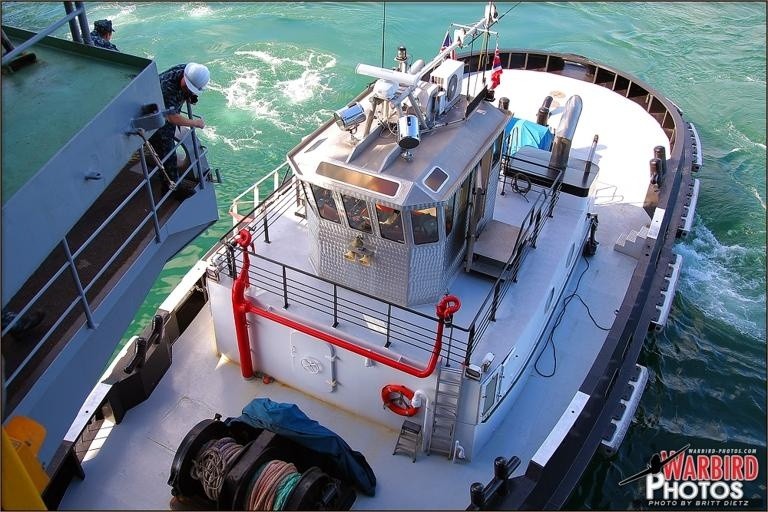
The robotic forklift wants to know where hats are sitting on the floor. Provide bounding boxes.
[94,19,115,32]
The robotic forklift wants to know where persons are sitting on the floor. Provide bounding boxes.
[1,308,47,342]
[144,61,211,201]
[89,18,121,53]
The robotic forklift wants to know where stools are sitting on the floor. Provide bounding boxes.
[392,420,422,463]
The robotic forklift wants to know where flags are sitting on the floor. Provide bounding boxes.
[489,44,503,91]
[439,31,459,60]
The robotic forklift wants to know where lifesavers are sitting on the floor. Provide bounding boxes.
[381,384,422,418]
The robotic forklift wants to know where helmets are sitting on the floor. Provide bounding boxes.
[184,62,210,94]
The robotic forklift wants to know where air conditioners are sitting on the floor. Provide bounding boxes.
[429,59,465,114]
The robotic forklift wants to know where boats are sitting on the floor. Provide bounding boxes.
[41,1,703,511]
[0,2,224,474]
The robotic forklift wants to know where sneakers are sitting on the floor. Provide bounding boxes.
[161,184,197,199]
